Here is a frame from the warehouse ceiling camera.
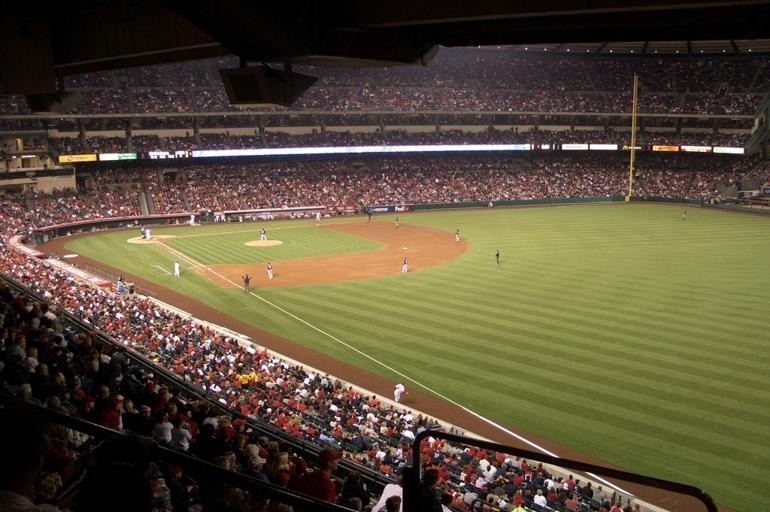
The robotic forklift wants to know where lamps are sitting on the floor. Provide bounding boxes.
[215,52,322,112]
[24,72,87,116]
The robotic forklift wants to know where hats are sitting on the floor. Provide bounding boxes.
[248,456,266,465]
[318,448,343,460]
[233,419,246,425]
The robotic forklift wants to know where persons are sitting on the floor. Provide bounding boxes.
[0,51,769,512]
